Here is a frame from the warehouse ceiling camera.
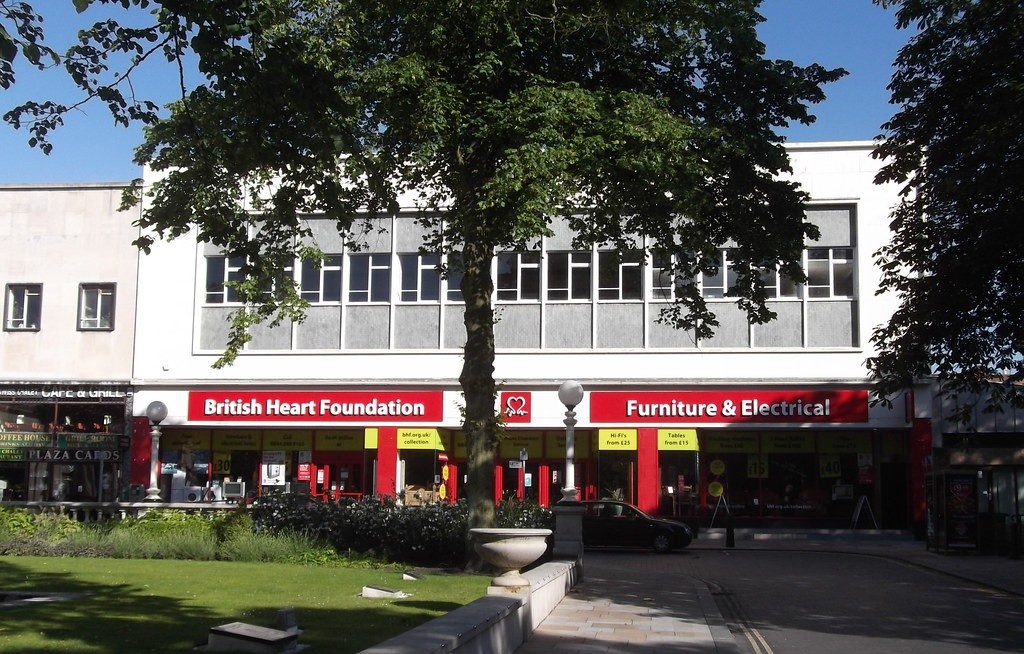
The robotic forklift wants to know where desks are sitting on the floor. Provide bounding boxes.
[670,494,698,516]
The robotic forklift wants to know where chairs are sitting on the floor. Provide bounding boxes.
[678,484,693,516]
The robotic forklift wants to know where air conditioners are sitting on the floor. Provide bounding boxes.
[183,485,202,503]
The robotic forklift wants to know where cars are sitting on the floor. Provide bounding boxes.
[555,500,694,551]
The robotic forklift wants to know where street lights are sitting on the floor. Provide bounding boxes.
[144,400,170,502]
[558,379,581,505]
